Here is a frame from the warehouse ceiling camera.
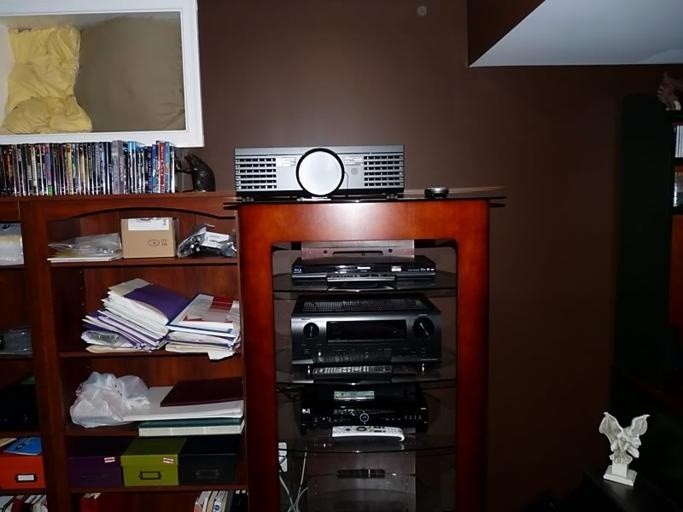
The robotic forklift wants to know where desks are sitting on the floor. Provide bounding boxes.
[529,463,682,512]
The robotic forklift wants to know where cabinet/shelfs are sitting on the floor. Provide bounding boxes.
[608,93,683,507]
[18,192,250,511]
[0,195,49,511]
[241,198,488,512]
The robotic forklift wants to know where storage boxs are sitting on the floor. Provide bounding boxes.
[119,437,187,486]
[119,216,176,259]
[68,436,134,490]
[178,434,240,487]
[0,441,47,492]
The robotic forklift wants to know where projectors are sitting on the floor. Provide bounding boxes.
[234,145,405,202]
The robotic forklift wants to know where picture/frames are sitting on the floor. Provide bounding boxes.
[0,0,205,147]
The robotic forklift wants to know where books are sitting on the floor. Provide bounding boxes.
[193,489,234,512]
[47,242,123,263]
[126,378,245,436]
[78,278,242,357]
[0,491,101,512]
[674,123,683,209]
[1,140,194,194]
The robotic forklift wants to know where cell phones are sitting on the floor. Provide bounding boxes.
[425,186,449,197]
